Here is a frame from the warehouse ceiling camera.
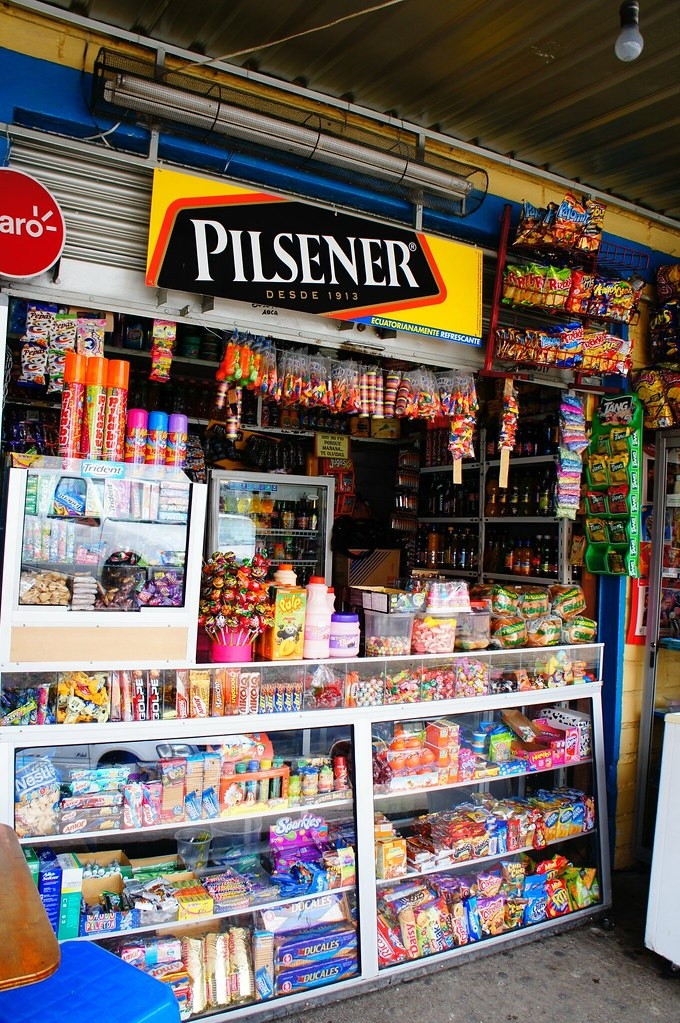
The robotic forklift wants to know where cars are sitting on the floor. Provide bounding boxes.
[15,733,274,788]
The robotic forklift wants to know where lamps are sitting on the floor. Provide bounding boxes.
[89,48,493,217]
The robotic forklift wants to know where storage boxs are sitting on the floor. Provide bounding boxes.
[332,548,401,585]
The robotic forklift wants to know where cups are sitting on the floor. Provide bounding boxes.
[209,818,262,873]
[173,827,215,873]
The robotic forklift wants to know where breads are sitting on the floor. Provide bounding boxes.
[469,580,597,648]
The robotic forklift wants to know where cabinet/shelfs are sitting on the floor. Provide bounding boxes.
[1,294,402,527]
[1,639,616,1022]
[412,406,579,586]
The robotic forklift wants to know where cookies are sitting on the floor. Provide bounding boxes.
[159,757,222,823]
[182,928,276,1013]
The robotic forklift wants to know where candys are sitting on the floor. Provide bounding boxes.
[199,550,275,635]
[365,619,455,655]
[350,659,519,707]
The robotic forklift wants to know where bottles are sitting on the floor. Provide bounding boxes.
[10,313,258,466]
[223,488,318,588]
[404,573,445,594]
[303,576,331,659]
[326,586,337,614]
[221,755,350,802]
[414,410,580,579]
[261,399,370,438]
